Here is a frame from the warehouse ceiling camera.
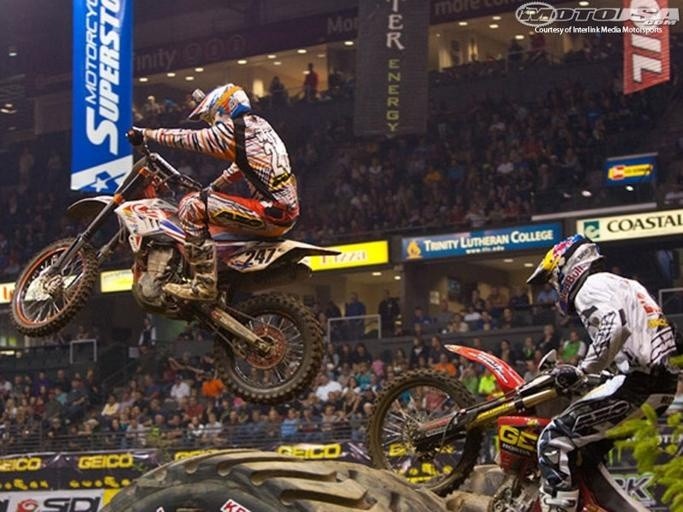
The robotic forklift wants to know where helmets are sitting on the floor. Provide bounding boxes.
[525,235,606,319]
[188,82,253,124]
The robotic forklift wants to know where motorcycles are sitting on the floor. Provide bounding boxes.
[359,340,681,512]
[8,126,341,406]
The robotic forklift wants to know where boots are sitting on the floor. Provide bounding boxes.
[164,243,220,302]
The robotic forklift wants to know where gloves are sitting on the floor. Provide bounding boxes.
[129,126,148,147]
[551,365,585,389]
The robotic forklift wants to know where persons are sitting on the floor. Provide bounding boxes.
[0,31,683,512]
